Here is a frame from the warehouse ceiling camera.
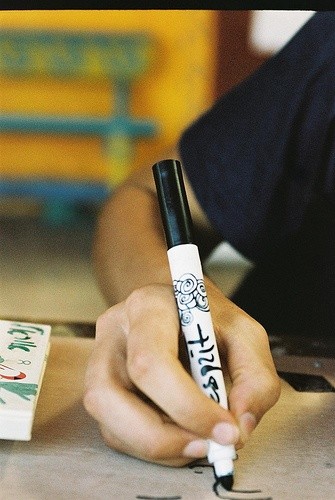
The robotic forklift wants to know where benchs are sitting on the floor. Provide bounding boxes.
[1,30,156,228]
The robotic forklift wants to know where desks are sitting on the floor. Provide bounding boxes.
[0,315,335,500]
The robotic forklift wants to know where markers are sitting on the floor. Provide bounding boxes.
[152,159,235,490]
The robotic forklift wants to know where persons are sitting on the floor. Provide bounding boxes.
[82,11,335,467]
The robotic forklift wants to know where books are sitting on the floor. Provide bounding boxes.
[0,319,52,442]
